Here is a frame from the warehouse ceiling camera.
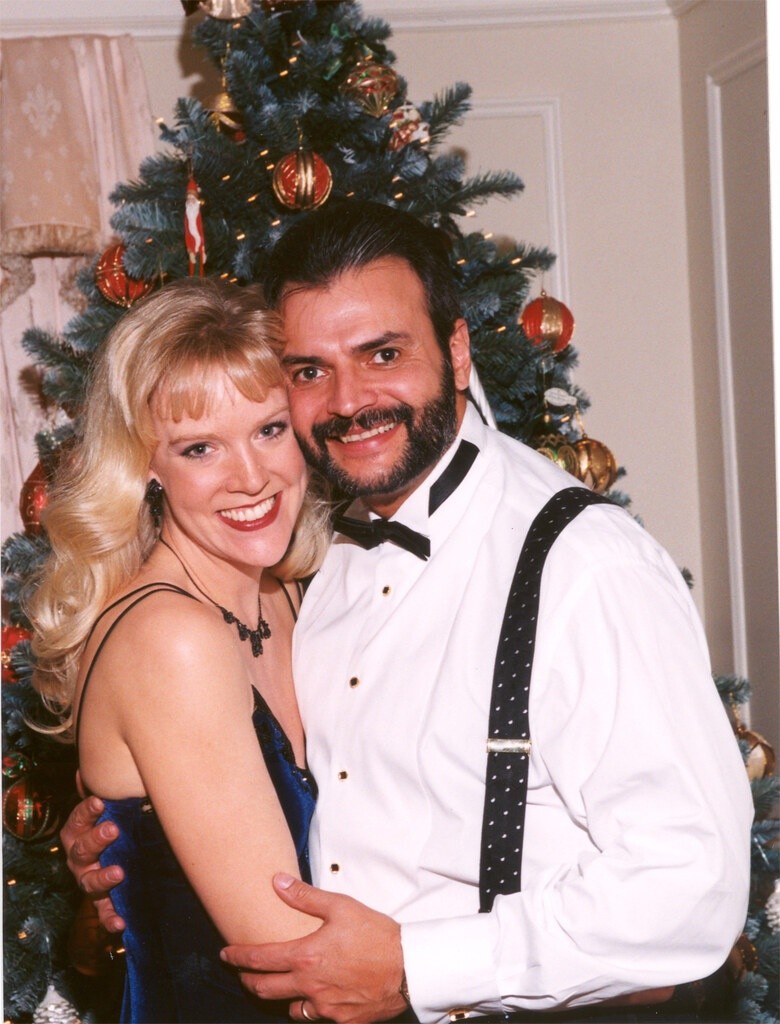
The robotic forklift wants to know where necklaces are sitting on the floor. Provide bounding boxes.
[159,532,272,657]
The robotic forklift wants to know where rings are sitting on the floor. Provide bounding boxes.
[301,999,318,1022]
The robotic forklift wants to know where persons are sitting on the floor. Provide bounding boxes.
[27,288,676,1024]
[59,197,754,1024]
